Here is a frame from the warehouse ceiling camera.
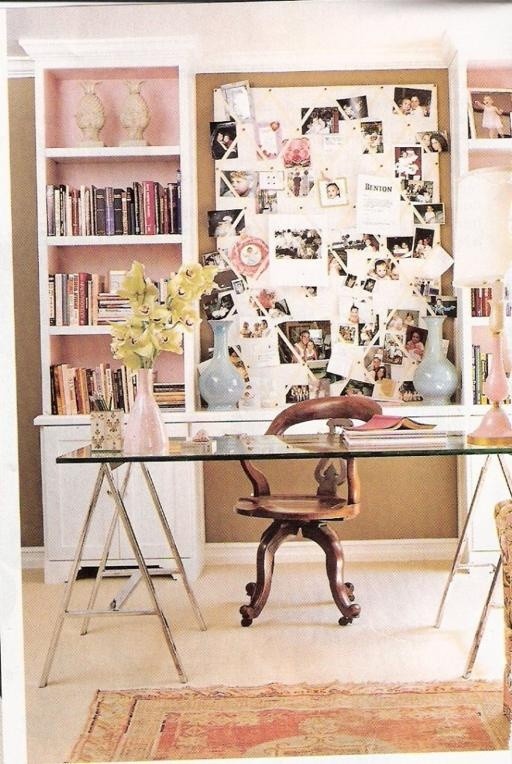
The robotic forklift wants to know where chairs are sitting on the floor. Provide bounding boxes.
[234,396,387,626]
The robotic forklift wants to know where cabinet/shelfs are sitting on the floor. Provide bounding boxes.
[448,49,512,573]
[36,58,206,583]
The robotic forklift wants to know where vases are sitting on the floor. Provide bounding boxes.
[413,315,459,405]
[120,367,168,452]
[199,319,244,411]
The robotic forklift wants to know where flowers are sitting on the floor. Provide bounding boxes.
[105,259,219,375]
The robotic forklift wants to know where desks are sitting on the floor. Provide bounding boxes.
[39,432,511,684]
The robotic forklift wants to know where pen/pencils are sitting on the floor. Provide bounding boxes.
[88,390,112,411]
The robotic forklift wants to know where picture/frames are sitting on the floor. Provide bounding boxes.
[467,87,512,138]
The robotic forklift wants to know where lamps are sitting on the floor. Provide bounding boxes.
[452,164,511,445]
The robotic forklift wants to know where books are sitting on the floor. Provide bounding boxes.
[342,429,454,443]
[472,286,510,405]
[343,413,439,432]
[44,176,184,414]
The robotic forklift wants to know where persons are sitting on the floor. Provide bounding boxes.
[474,95,507,139]
[196,85,456,408]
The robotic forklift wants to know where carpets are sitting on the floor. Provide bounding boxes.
[67,684,509,762]
